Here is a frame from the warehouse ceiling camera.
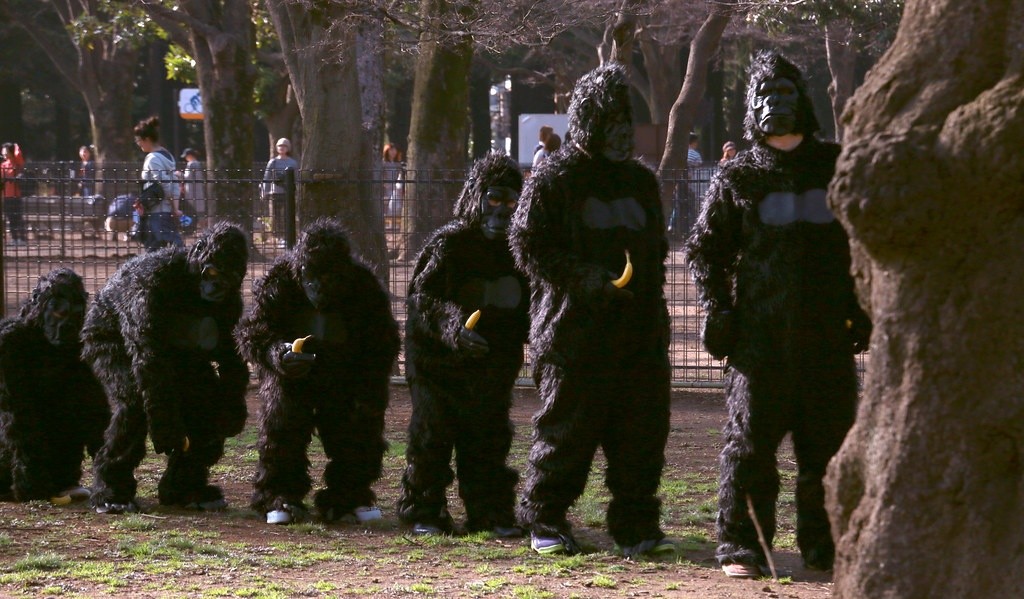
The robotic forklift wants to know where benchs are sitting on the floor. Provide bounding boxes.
[2,193,106,239]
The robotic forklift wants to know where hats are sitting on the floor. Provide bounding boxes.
[275,138,291,150]
[180,147,194,157]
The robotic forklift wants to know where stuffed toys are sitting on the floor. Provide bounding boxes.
[0,269,110,502]
[682,54,872,580]
[395,152,525,537]
[508,65,676,556]
[234,218,399,527]
[82,224,249,516]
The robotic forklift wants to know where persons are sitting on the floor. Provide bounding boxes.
[133,115,183,254]
[105,192,139,240]
[719,141,737,169]
[0,142,26,242]
[381,142,406,243]
[261,138,299,240]
[668,132,703,235]
[18,181,93,238]
[532,125,571,173]
[77,146,95,239]
[174,149,205,235]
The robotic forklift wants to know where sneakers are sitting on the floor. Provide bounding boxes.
[412,522,446,536]
[720,560,760,577]
[495,527,523,537]
[625,539,676,555]
[531,530,568,555]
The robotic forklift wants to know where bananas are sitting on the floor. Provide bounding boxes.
[292,335,312,353]
[609,250,633,288]
[464,309,480,330]
[49,494,72,506]
[183,436,190,451]
